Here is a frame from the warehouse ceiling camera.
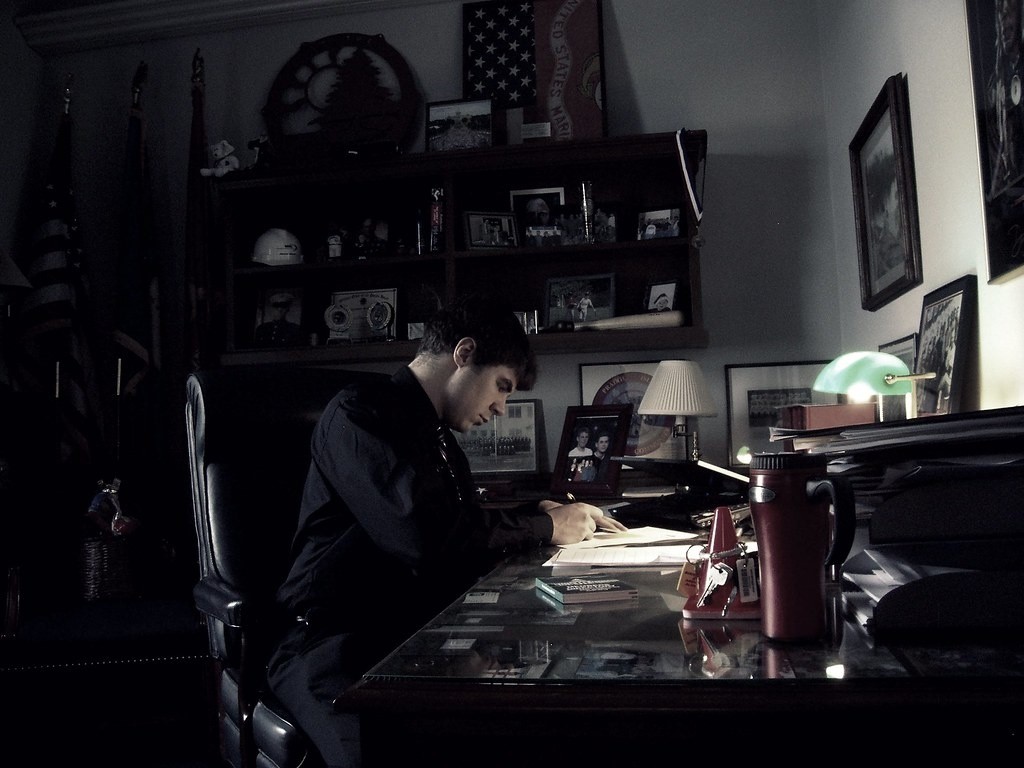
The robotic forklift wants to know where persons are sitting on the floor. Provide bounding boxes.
[479,219,501,244]
[525,197,560,242]
[918,307,958,414]
[568,429,610,481]
[650,294,671,312]
[266,287,628,768]
[256,294,299,346]
[638,216,679,238]
[557,288,596,322]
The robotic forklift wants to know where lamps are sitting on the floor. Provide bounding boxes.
[814,352,937,424]
[635,360,752,482]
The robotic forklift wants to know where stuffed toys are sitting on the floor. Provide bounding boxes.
[200,140,239,177]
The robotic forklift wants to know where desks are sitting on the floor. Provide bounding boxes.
[327,514,1021,768]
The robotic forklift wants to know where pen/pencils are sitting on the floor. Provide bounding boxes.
[566,492,600,532]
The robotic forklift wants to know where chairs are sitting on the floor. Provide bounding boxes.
[0,318,215,767]
[178,365,386,766]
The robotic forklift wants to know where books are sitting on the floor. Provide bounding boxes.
[536,572,638,603]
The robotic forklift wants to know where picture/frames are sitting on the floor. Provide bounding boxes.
[462,210,523,252]
[645,278,680,313]
[846,71,923,314]
[425,96,495,153]
[547,403,634,498]
[724,360,832,473]
[447,398,545,479]
[911,274,978,418]
[543,271,616,328]
[578,360,690,462]
[509,187,565,212]
[878,332,919,423]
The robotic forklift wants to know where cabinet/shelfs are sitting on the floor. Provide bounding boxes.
[212,129,708,373]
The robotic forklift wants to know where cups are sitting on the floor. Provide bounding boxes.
[749,454,856,642]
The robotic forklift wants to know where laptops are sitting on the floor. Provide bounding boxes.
[608,456,755,529]
